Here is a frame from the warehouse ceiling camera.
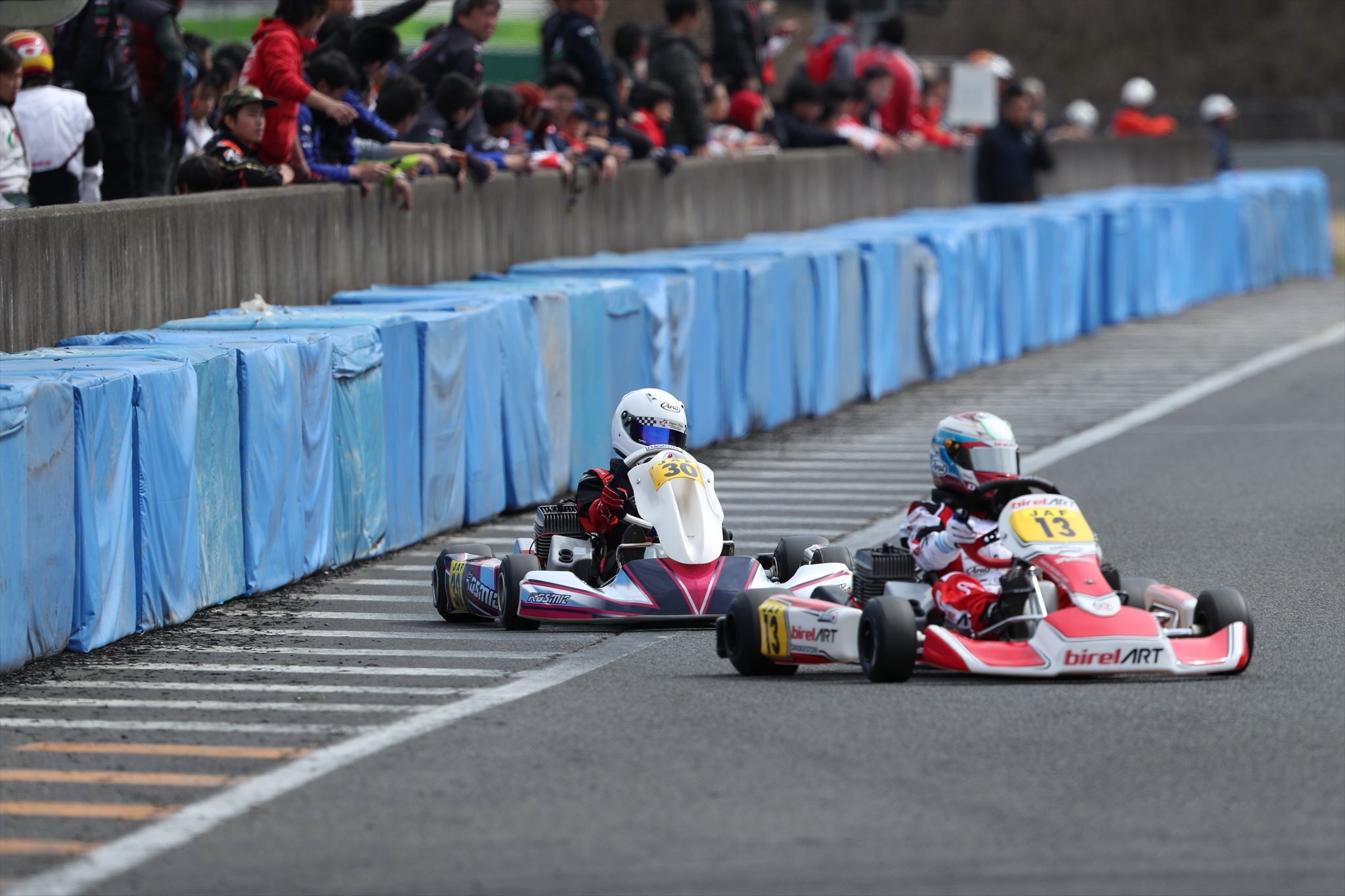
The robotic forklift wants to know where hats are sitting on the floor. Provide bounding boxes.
[218,85,280,113]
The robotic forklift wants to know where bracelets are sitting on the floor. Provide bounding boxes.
[387,172,408,187]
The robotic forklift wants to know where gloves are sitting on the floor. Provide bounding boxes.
[945,508,978,548]
[601,485,628,510]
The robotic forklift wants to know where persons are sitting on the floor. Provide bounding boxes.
[908,412,1120,642]
[574,388,734,589]
[0,0,1235,209]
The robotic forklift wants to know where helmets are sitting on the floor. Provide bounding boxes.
[930,411,1020,500]
[969,50,1016,82]
[4,29,52,77]
[1065,100,1098,135]
[1122,77,1156,109]
[611,388,688,467]
[1199,94,1235,120]
